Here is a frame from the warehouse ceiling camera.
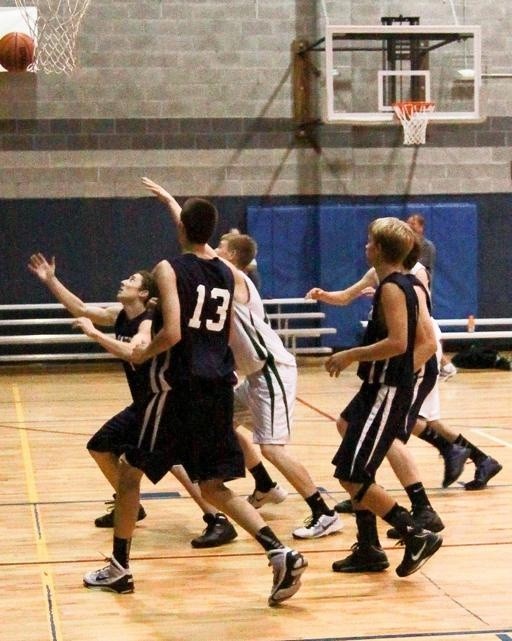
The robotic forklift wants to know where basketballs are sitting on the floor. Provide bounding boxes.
[0,33,37,72]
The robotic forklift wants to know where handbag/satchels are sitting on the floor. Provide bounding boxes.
[452,342,497,369]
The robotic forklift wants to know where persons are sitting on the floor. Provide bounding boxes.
[406,212,437,273]
[304,221,504,490]
[324,215,445,579]
[137,176,344,541]
[25,250,238,549]
[333,242,444,538]
[82,196,309,608]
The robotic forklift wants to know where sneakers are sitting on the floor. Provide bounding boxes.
[83,552,134,594]
[191,513,237,547]
[332,500,444,577]
[95,494,146,527]
[246,482,288,509]
[465,456,502,490]
[443,444,472,487]
[267,546,308,607]
[293,511,344,539]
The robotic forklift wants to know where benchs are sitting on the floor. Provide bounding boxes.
[0,297,512,361]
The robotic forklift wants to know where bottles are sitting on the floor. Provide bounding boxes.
[467,315,475,333]
